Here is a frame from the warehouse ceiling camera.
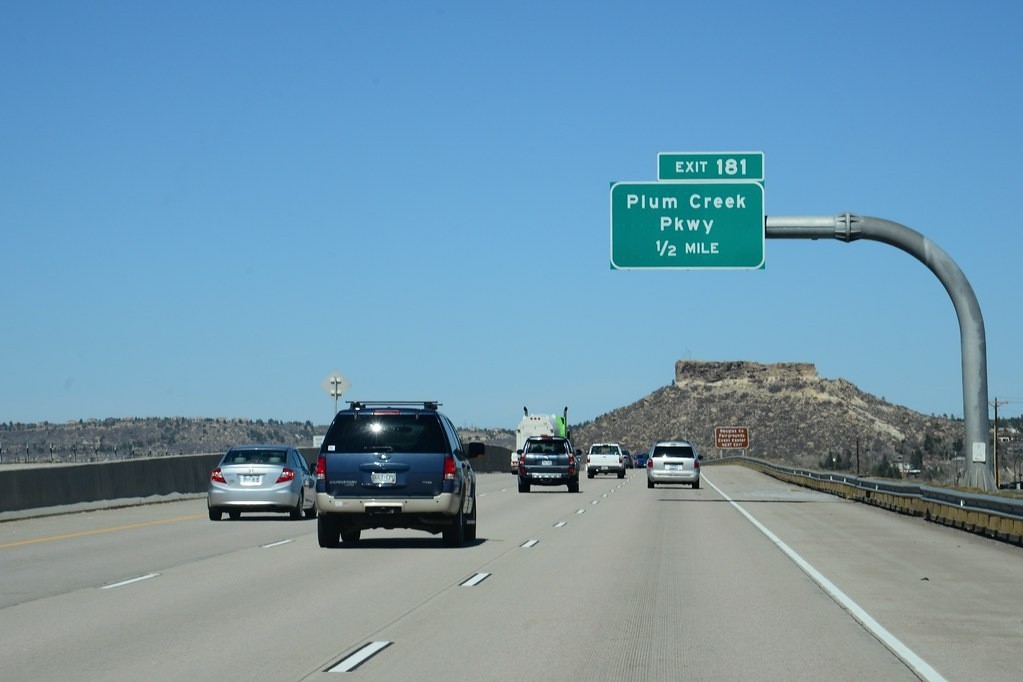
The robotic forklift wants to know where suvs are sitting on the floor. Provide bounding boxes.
[517,434,583,493]
[312,399,486,549]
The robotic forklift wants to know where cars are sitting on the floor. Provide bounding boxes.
[622,450,649,469]
[643,439,704,489]
[205,444,318,521]
[584,441,628,479]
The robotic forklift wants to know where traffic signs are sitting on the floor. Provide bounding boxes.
[607,150,767,271]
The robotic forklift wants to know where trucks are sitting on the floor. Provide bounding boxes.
[510,406,571,475]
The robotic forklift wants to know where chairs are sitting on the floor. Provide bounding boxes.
[532,446,542,452]
[269,458,281,463]
[555,446,565,454]
[544,449,552,452]
[235,457,246,464]
